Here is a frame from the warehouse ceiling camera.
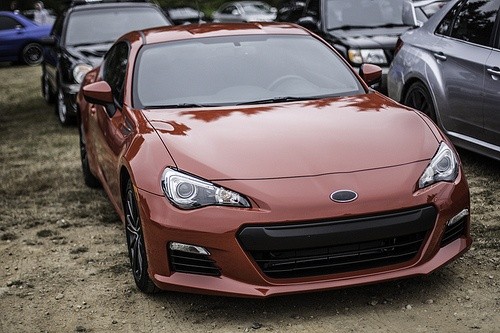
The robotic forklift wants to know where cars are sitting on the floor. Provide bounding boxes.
[78,22,472,299]
[40,2,176,128]
[387,1,500,161]
[168,2,277,24]
[0,11,53,67]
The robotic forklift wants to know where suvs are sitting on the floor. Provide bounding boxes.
[277,0,420,88]
